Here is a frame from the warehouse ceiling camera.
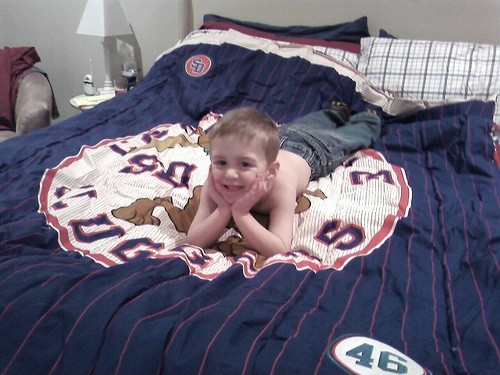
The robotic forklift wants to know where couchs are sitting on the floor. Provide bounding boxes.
[0,46,60,142]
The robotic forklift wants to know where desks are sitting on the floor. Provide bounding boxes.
[69,94,116,112]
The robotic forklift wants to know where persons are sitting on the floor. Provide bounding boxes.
[187,102,381,250]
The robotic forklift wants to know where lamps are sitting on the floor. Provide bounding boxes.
[76,0,133,94]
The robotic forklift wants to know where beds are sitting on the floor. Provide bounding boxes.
[0,14,500,375]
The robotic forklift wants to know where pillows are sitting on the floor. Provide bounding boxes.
[357,37,500,115]
[184,30,360,69]
